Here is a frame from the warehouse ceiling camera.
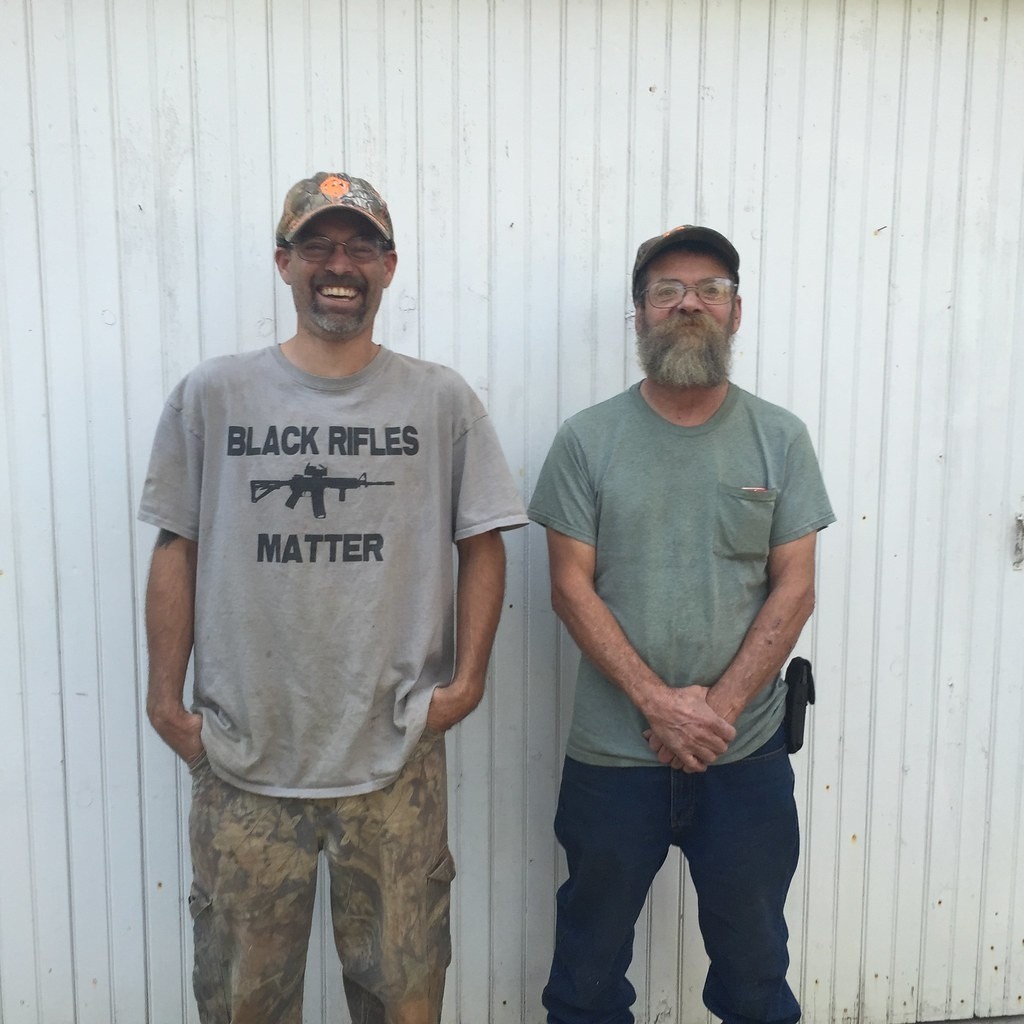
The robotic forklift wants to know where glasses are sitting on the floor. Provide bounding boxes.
[288,235,388,262]
[637,277,737,309]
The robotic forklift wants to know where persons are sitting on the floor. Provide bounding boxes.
[135,171,528,1024]
[527,223,840,1024]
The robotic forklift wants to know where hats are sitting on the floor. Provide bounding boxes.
[275,170,394,250]
[631,225,741,295]
